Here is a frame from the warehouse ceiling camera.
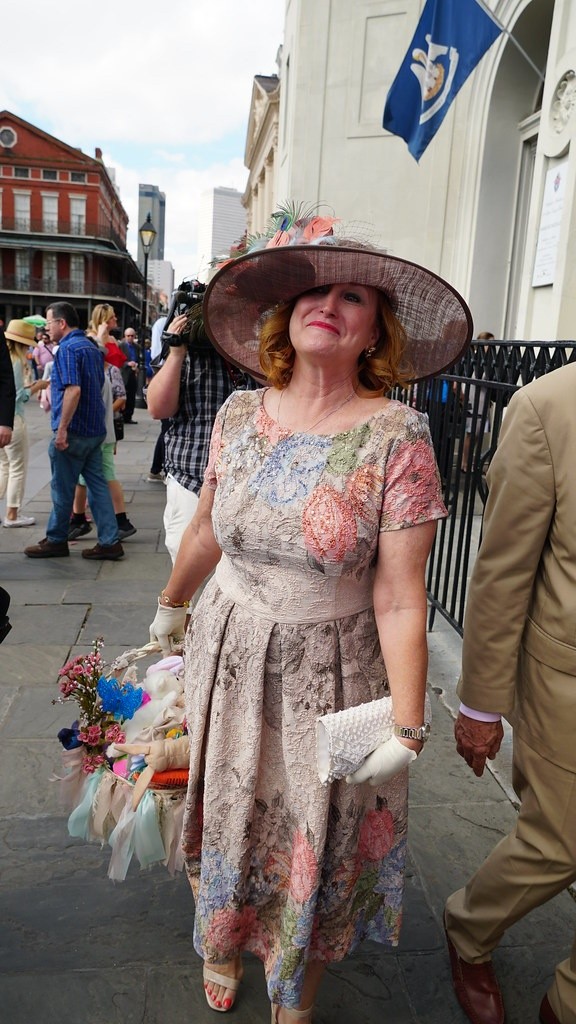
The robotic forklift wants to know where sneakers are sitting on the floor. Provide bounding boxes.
[25,536,70,557]
[82,543,124,559]
[118,520,136,540]
[68,519,92,541]
[148,473,163,482]
[4,515,36,527]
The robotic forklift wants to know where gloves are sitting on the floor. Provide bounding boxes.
[149,597,187,659]
[345,735,422,788]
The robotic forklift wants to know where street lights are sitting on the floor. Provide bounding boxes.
[134,211,157,408]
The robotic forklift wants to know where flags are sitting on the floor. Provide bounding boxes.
[379,0,501,163]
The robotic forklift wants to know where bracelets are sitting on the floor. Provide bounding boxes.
[161,589,191,610]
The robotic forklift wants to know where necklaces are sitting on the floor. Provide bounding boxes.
[276,387,355,431]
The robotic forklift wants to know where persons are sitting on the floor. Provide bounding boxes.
[148,231,474,1024]
[440,360,576,1024]
[394,332,496,481]
[0,277,263,646]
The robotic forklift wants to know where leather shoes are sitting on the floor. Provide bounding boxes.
[443,908,506,1024]
[539,993,560,1024]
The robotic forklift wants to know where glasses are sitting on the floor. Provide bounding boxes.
[102,303,109,311]
[127,334,134,338]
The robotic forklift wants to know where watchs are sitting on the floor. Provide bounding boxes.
[392,723,431,742]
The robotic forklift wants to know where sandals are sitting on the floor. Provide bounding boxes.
[270,1000,314,1024]
[203,964,241,1011]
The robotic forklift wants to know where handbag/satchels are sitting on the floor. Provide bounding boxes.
[114,418,124,440]
[314,693,431,787]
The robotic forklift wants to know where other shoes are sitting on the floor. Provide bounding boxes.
[124,418,138,424]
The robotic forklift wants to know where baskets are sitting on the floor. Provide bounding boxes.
[101,642,189,799]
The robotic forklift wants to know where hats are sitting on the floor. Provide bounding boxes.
[4,319,38,347]
[201,200,473,390]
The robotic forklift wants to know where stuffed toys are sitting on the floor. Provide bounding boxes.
[105,655,191,812]
